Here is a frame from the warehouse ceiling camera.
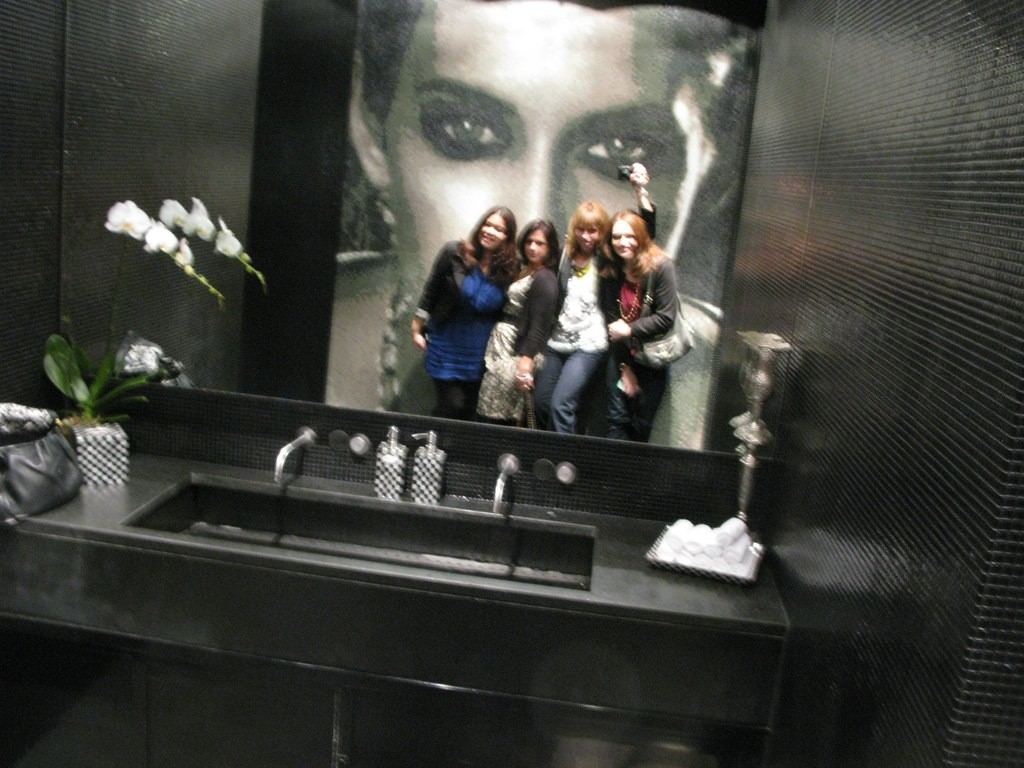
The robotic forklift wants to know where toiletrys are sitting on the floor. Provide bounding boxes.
[373,426,409,501]
[410,430,448,505]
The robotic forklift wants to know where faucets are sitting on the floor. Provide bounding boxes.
[273,428,315,483]
[492,453,517,516]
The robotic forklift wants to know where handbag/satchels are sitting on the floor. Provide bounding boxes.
[640,262,696,367]
[0,402,83,526]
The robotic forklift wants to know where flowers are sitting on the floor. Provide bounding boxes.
[43,201,229,423]
[63,195,263,383]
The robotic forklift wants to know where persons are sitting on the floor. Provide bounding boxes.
[592,209,681,444]
[475,218,560,428]
[411,206,520,420]
[325,0,756,451]
[534,163,658,434]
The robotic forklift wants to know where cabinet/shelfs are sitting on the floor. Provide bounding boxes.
[0,519,784,768]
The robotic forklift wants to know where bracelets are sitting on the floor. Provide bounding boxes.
[515,372,534,382]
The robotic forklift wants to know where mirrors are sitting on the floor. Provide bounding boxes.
[59,0,840,469]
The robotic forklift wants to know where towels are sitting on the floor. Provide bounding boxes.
[655,516,754,576]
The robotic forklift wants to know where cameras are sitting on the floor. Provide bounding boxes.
[618,165,634,180]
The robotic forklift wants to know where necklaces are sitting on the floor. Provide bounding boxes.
[617,281,640,322]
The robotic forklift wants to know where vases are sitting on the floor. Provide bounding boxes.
[58,420,128,489]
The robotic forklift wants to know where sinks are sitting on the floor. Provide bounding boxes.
[115,468,600,597]
